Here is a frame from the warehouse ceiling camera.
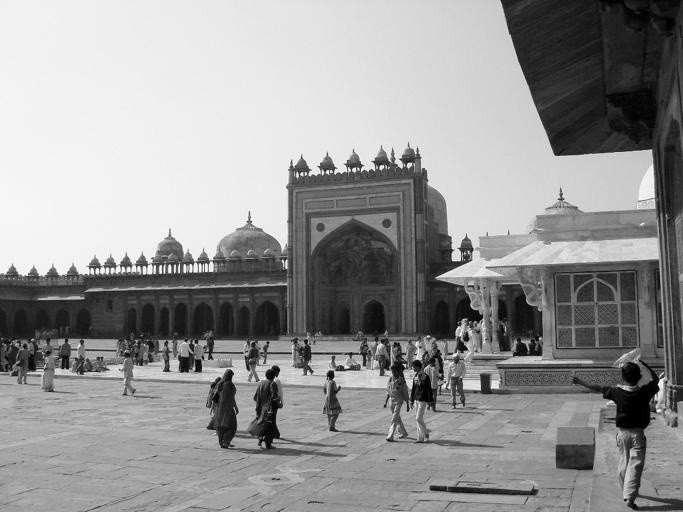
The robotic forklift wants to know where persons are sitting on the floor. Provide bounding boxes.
[446,353,467,408]
[383,364,409,441]
[212,369,239,447]
[406,334,450,392]
[352,329,365,341]
[512,337,542,357]
[360,336,402,370]
[118,350,137,395]
[178,337,205,372]
[1,336,105,393]
[454,318,511,363]
[243,337,269,382]
[569,358,658,509]
[291,338,314,375]
[247,369,281,448]
[206,330,216,360]
[409,360,436,443]
[323,370,342,430]
[328,355,344,371]
[346,353,361,371]
[163,340,173,372]
[306,330,317,345]
[116,332,155,366]
